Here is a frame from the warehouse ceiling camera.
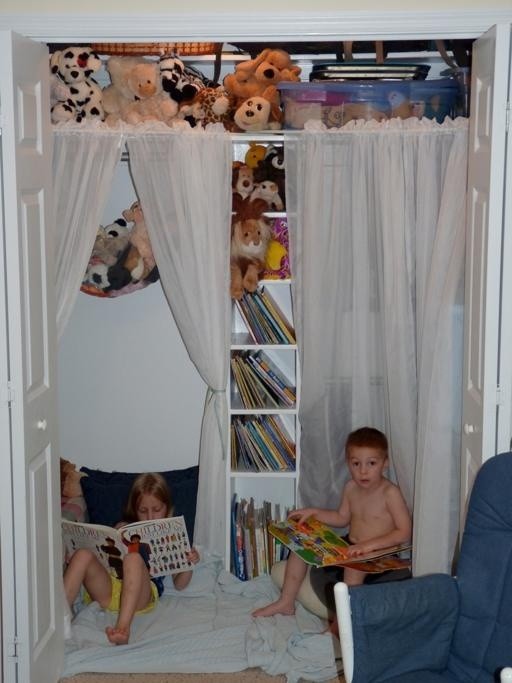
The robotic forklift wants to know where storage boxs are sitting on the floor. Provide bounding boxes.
[276,77,461,132]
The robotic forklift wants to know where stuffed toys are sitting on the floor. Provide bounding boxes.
[49,46,450,132]
[82,201,160,292]
[50,46,450,297]
[229,142,291,297]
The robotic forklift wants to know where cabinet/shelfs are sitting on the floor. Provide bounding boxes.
[226,136,302,580]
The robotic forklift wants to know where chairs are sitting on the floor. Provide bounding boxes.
[333,449,511,681]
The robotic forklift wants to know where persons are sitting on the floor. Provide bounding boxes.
[252,427,413,638]
[100,538,123,581]
[64,470,201,644]
[121,530,153,575]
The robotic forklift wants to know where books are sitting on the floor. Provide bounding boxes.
[230,285,296,582]
[62,516,199,582]
[268,518,413,575]
